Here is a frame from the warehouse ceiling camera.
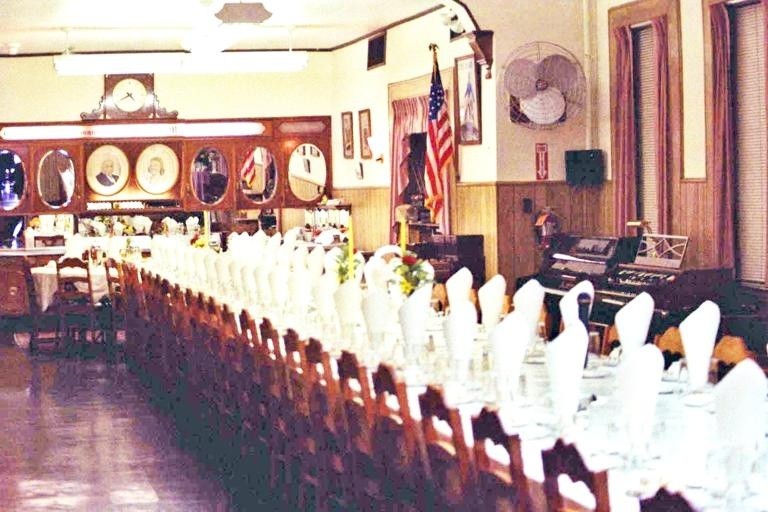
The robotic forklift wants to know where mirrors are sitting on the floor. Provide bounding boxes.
[0,148,76,212]
[190,143,328,206]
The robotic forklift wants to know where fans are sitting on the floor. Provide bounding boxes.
[496,41,587,132]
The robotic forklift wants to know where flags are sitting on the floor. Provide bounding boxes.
[240,148,257,189]
[420,51,455,225]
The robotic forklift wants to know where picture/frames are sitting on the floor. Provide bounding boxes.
[341,108,373,160]
[454,53,483,145]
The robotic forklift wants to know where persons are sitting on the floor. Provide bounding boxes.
[144,155,170,188]
[95,158,120,187]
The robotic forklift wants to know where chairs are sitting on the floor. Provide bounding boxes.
[19,228,768,511]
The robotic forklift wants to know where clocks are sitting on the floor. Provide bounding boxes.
[102,72,156,120]
